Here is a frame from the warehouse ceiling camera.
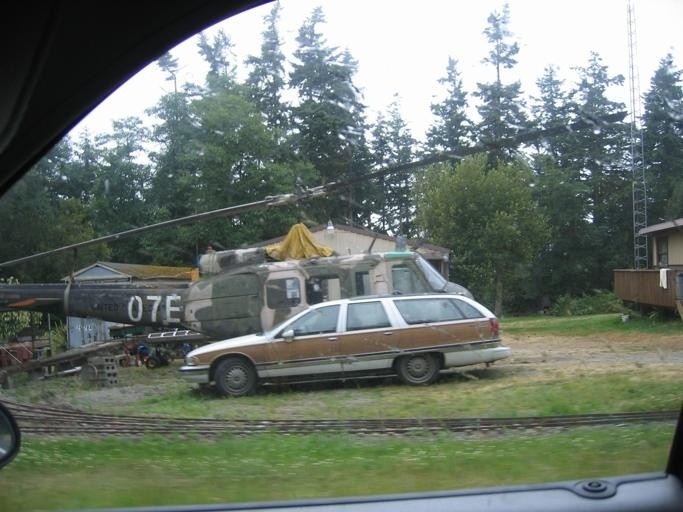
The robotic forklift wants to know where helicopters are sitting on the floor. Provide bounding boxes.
[1,113,627,366]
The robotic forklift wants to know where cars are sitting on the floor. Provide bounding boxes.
[180,292,511,400]
